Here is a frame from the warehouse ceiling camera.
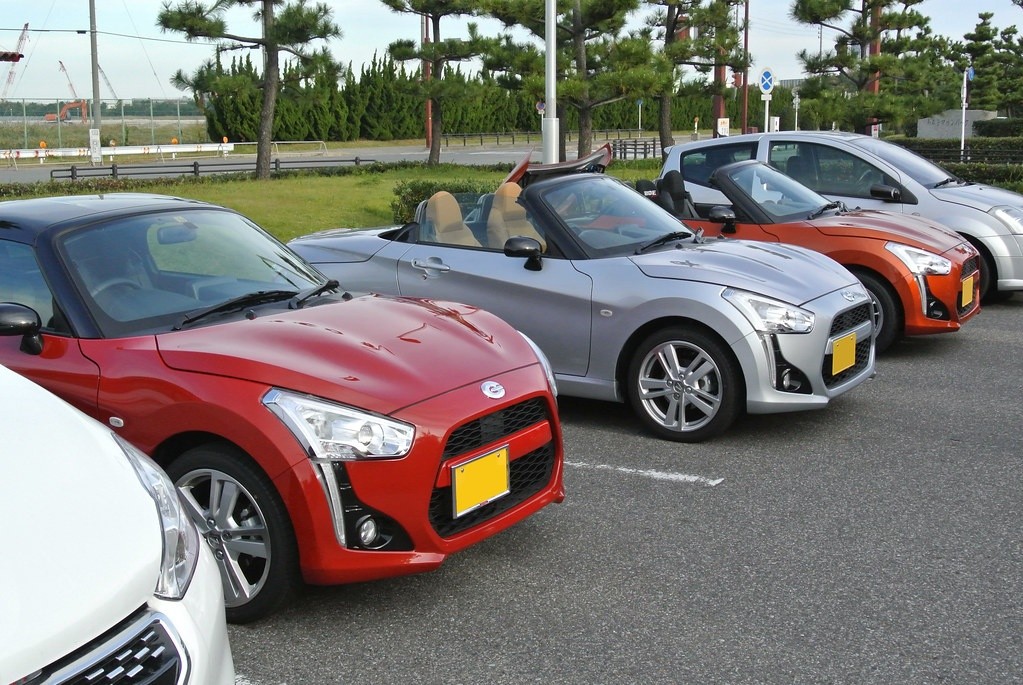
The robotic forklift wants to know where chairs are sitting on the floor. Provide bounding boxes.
[786,156,806,177]
[488,182,547,253]
[636,170,685,216]
[426,191,483,247]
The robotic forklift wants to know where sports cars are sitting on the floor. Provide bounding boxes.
[279,169,883,443]
[572,155,983,352]
[0,193,570,627]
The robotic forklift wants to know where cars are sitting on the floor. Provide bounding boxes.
[649,129,1023,306]
[0,359,238,685]
